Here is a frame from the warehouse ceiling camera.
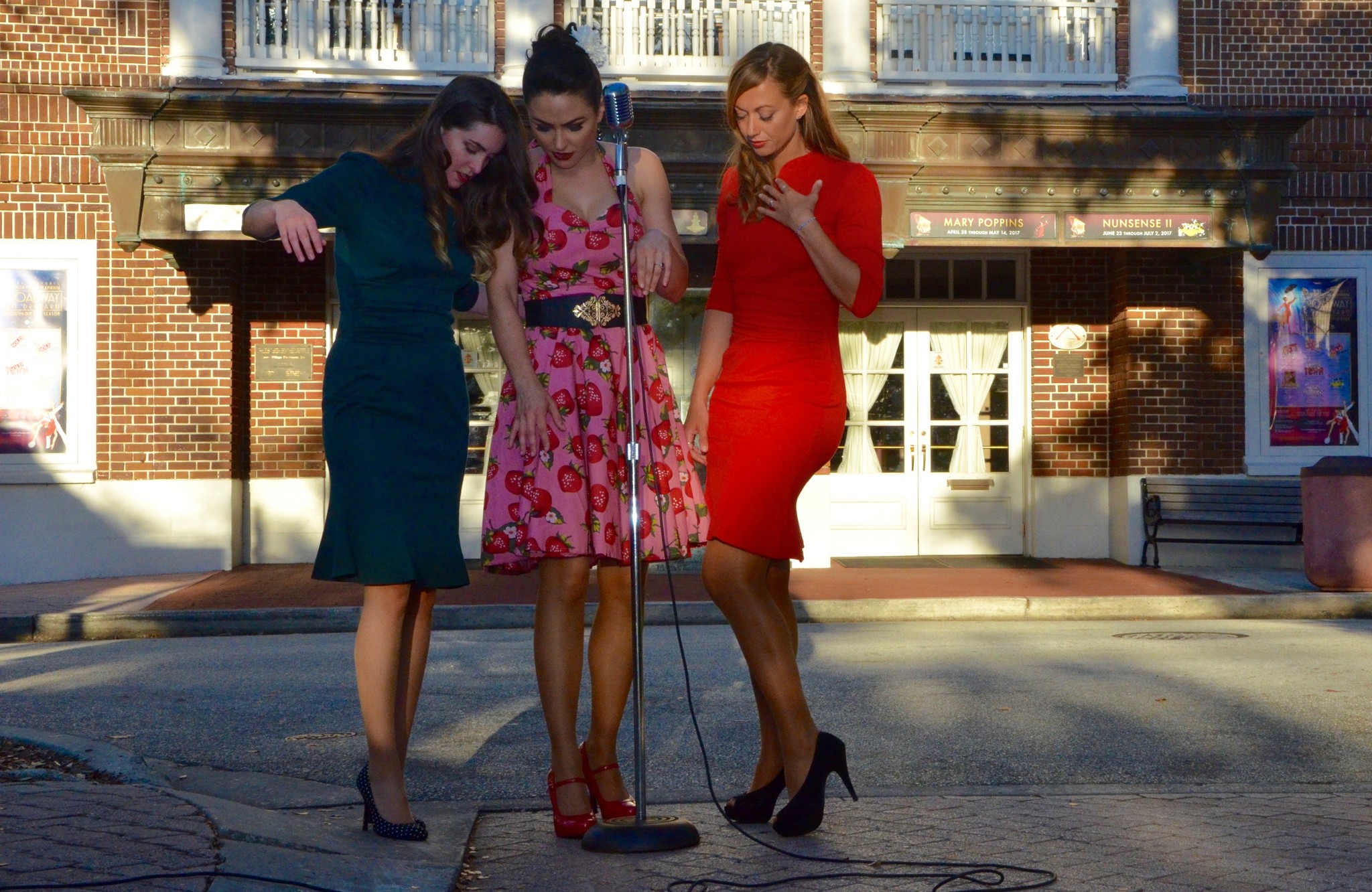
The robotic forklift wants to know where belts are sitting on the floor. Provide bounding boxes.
[522,293,649,331]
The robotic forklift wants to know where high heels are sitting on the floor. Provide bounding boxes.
[577,741,637,820]
[724,767,787,824]
[547,766,599,838]
[773,733,858,839]
[357,765,428,842]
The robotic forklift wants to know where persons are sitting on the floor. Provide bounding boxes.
[243,75,546,841]
[680,40,885,843]
[482,23,711,838]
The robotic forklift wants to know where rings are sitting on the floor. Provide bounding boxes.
[781,184,788,190]
[768,200,774,207]
[654,262,664,269]
[688,442,693,444]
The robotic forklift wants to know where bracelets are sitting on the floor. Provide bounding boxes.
[795,216,815,234]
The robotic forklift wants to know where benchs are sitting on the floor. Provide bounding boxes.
[1140,476,1304,570]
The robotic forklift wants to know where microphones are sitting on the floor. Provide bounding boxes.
[601,82,634,198]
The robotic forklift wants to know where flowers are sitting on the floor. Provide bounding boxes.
[569,25,608,67]
